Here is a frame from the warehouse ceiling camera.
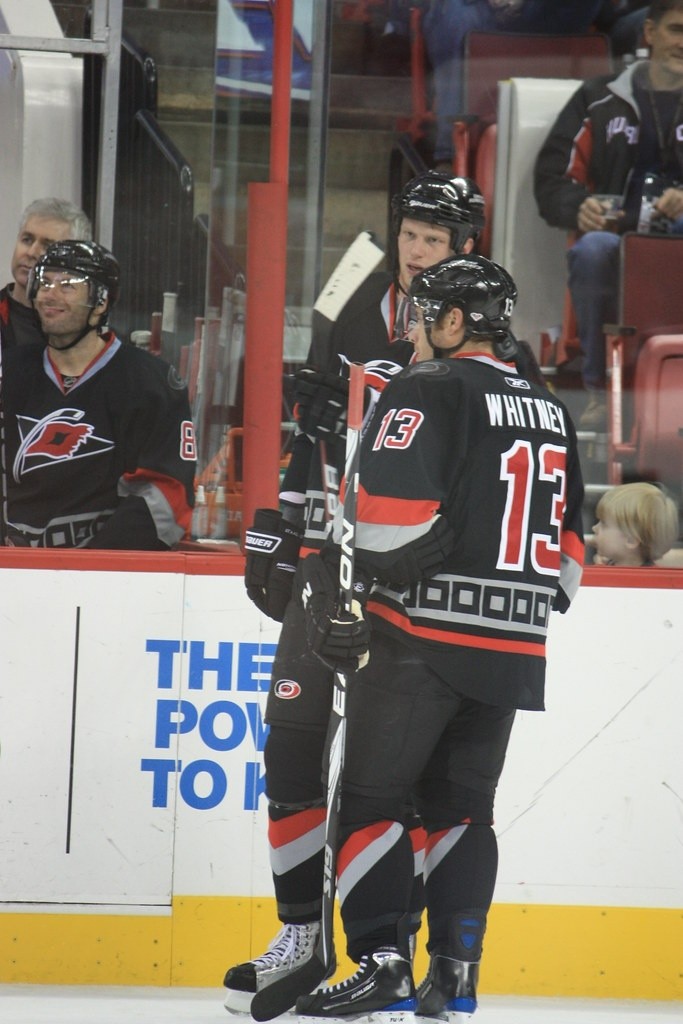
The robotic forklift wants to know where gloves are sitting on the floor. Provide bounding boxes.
[365,516,466,587]
[292,370,381,447]
[291,552,374,676]
[244,508,306,623]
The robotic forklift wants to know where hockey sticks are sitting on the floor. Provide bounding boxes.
[249,364,366,1022]
[141,280,247,482]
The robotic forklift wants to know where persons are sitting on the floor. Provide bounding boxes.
[530,0,683,390]
[226,177,586,1024]
[0,199,198,550]
[588,481,680,566]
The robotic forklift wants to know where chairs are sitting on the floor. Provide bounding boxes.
[381,0,683,491]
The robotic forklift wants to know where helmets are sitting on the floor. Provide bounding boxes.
[408,253,519,335]
[28,239,123,317]
[390,168,487,252]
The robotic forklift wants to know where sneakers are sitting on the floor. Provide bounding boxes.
[223,917,337,1013]
[295,944,418,1024]
[415,951,480,1021]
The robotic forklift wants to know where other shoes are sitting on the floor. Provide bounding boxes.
[434,162,473,180]
[578,398,608,430]
[548,359,597,391]
